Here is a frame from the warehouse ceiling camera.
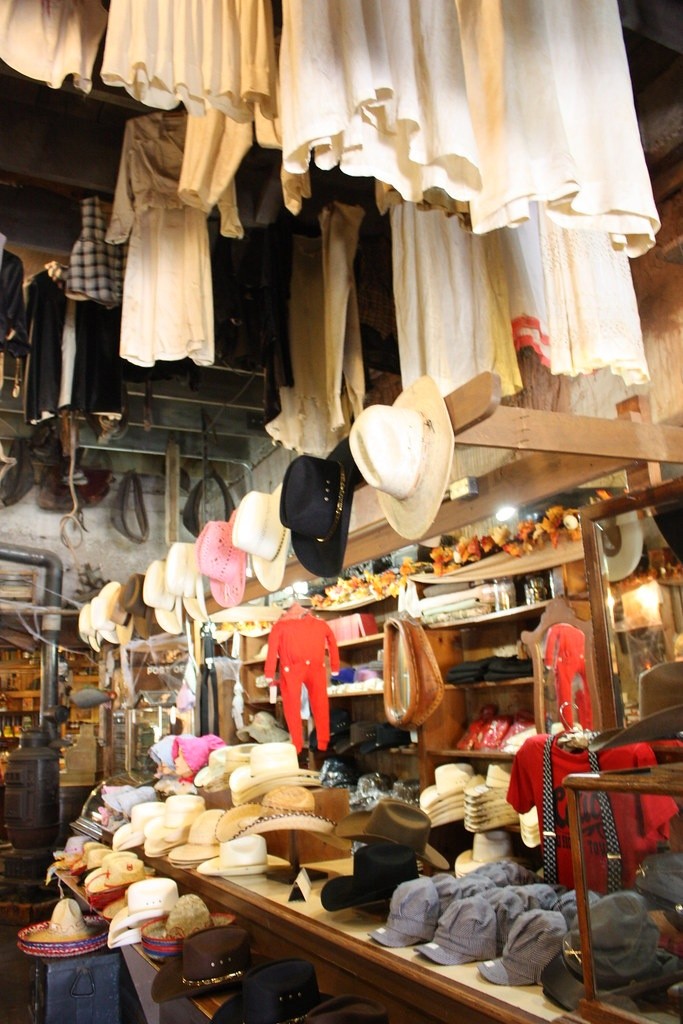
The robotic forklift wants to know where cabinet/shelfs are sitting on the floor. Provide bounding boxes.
[0,644,98,744]
[242,535,637,895]
[54,760,683,1024]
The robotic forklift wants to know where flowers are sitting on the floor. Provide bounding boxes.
[312,505,583,610]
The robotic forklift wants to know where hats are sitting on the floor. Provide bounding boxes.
[280,437,354,579]
[347,374,455,540]
[17,897,111,959]
[142,559,186,635]
[53,734,679,1024]
[118,574,153,640]
[77,581,134,652]
[164,542,208,621]
[232,484,291,591]
[194,512,245,608]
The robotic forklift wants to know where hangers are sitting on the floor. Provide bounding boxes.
[281,590,310,621]
